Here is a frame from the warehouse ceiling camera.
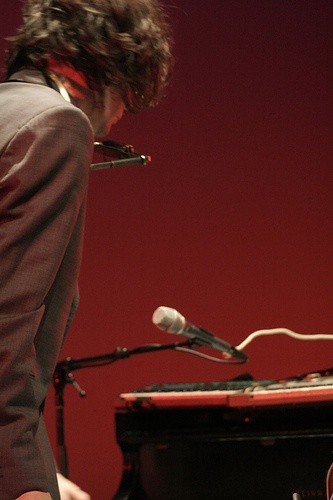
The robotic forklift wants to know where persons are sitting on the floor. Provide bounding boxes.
[0,0,169,500]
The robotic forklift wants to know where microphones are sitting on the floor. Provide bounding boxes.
[152,306,248,360]
